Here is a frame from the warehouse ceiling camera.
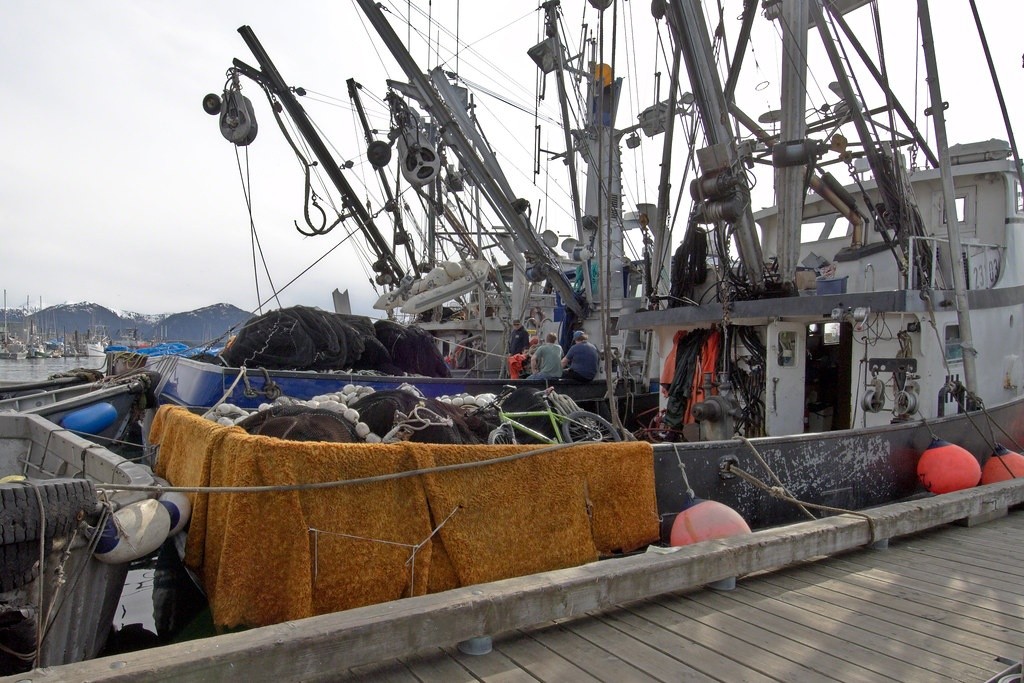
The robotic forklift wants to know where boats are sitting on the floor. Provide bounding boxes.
[396,126,441,187]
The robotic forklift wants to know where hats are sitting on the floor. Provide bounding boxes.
[573,331,584,340]
[513,319,520,324]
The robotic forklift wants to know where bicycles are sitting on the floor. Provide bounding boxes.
[464,384,623,445]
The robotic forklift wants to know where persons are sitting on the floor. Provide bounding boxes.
[508,318,599,381]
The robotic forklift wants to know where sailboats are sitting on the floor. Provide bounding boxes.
[0,0,1023,673]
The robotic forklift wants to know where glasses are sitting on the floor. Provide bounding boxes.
[514,324,519,326]
